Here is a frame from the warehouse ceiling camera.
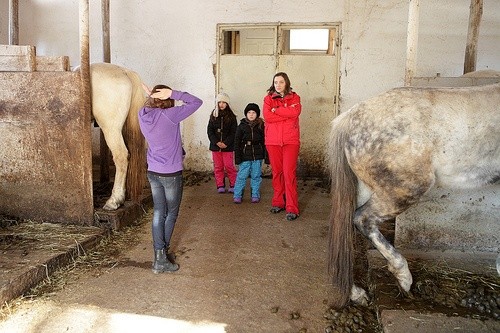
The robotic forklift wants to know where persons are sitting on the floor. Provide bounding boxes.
[233,103,270,203]
[138,85,203,273]
[207,91,237,193]
[262,72,302,220]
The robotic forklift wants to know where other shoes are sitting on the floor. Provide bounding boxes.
[234,197,241,203]
[252,197,260,203]
[286,212,298,221]
[218,188,226,193]
[228,187,234,193]
[270,206,284,213]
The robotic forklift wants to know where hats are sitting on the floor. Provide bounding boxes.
[212,93,237,118]
[244,103,260,118]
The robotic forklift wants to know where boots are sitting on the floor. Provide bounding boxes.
[152,247,180,274]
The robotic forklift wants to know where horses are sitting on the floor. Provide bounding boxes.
[71,62,147,211]
[322,82,500,313]
[463,69,499,78]
